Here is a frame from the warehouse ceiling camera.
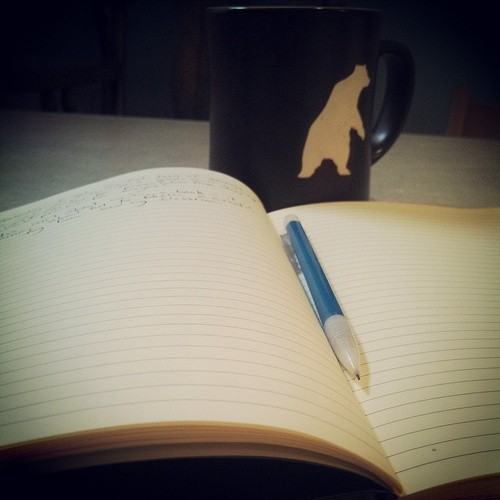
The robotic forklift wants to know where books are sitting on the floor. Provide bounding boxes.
[0,167,500,500]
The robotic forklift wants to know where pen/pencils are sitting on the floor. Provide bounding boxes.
[284,211,364,386]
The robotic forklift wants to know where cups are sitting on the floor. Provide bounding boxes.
[208,6,415,214]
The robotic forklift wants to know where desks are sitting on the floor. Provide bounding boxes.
[0,109,500,212]
